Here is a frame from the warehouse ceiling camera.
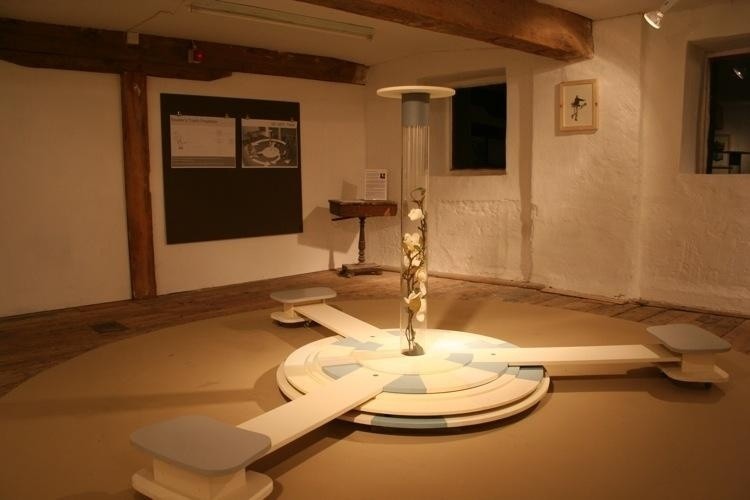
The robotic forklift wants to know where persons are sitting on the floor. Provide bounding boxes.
[570,95,585,121]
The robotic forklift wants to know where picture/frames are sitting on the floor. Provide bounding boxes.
[557,79,600,135]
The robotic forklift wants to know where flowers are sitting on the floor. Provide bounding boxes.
[401,184,430,356]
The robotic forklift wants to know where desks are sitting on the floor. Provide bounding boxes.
[329,198,398,280]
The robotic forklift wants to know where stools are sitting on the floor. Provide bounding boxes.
[269,287,338,326]
[648,320,733,394]
[128,413,276,499]
